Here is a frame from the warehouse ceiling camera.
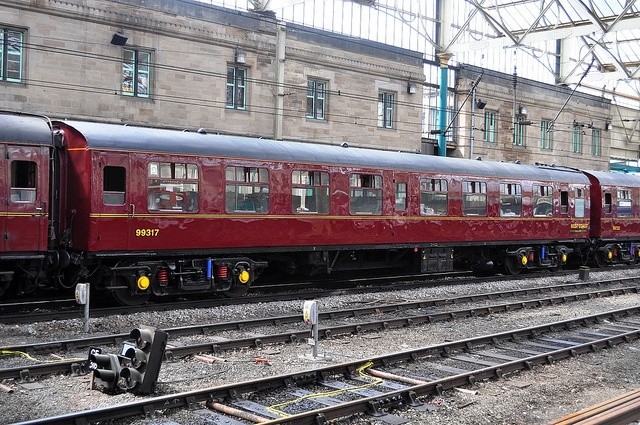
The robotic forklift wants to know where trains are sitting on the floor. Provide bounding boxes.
[1,111,640,294]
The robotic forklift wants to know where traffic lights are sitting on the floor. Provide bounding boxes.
[120,321,168,395]
[87,352,119,394]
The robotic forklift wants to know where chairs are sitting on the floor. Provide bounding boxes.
[175,192,188,209]
[151,191,175,209]
